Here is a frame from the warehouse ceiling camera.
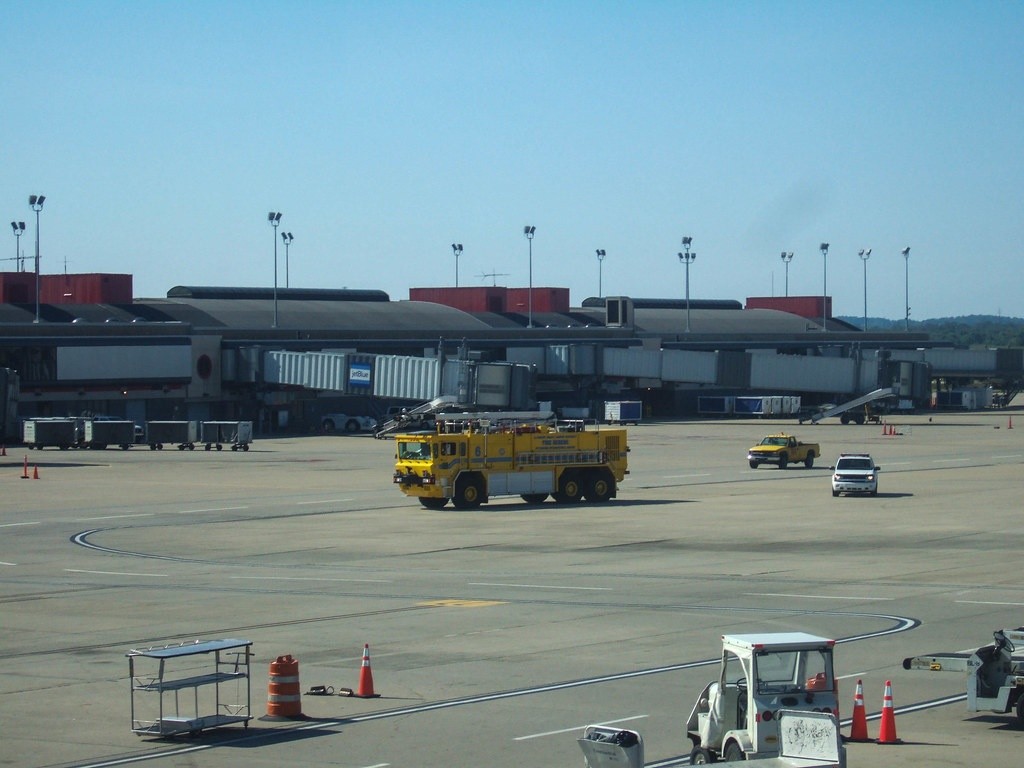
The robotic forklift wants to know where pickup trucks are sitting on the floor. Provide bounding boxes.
[746,433,821,470]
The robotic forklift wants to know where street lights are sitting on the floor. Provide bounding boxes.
[523,225,538,328]
[781,251,794,297]
[858,249,872,332]
[267,212,283,328]
[450,242,464,287]
[10,221,26,272]
[28,194,47,322]
[677,252,696,333]
[594,248,606,298]
[682,236,692,298]
[281,230,294,288]
[820,242,830,332]
[900,246,913,332]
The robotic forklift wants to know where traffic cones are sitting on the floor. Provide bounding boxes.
[352,642,381,698]
[874,680,902,745]
[33,464,41,480]
[0,443,8,456]
[844,679,874,743]
[891,425,896,436]
[888,424,892,435]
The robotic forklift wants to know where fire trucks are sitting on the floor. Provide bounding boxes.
[372,394,632,511]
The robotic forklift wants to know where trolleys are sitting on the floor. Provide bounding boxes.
[199,420,253,452]
[125,636,255,741]
[145,419,198,451]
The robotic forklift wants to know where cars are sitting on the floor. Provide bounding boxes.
[94,414,145,444]
[321,412,378,434]
[829,452,882,498]
